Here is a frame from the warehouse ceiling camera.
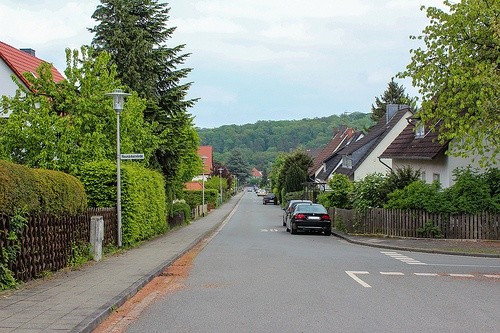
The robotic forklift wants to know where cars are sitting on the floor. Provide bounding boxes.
[247,188,252,192]
[281,200,313,226]
[263,193,277,205]
[253,186,269,197]
[286,203,332,236]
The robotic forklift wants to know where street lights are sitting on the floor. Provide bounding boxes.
[200,154,209,216]
[217,167,224,205]
[104,88,132,250]
